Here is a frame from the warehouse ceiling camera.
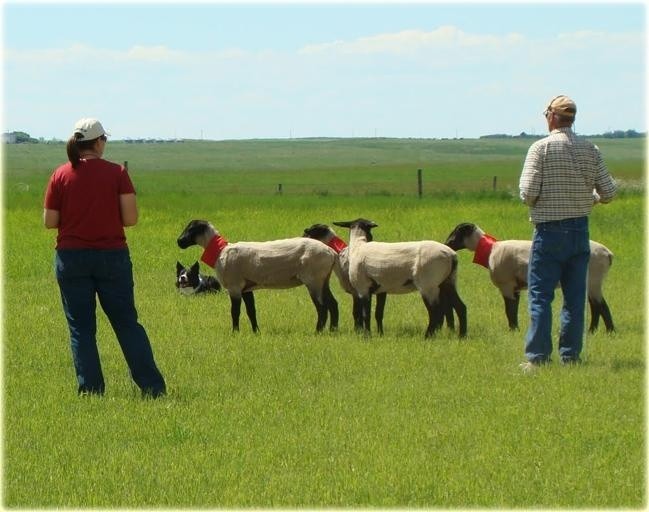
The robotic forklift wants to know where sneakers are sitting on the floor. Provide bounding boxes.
[519,360,546,370]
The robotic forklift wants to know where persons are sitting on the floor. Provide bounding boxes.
[518,94,617,368]
[44,118,167,400]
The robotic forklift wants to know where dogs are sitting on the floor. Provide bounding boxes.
[175,260,221,297]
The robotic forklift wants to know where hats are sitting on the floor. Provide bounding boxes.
[541,95,577,118]
[73,117,112,142]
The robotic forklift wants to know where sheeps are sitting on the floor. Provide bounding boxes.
[444,222,617,334]
[177,219,339,336]
[302,217,467,339]
